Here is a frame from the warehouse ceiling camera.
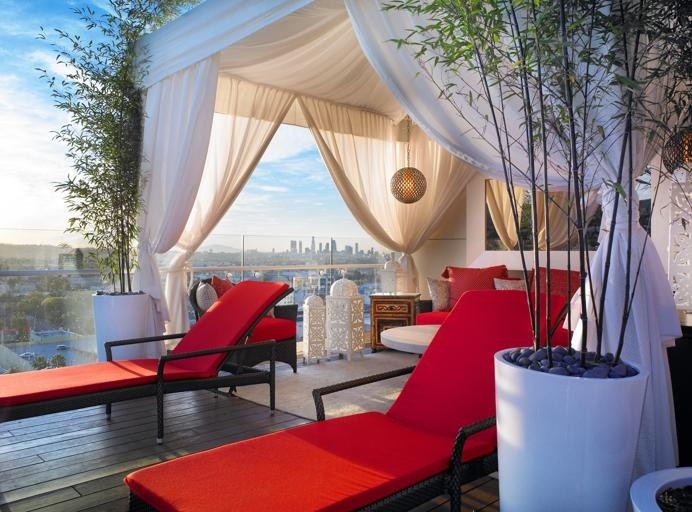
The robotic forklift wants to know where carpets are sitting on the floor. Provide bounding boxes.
[211,349,421,423]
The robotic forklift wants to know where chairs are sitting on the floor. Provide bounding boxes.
[121,287,570,511]
[189,277,300,394]
[0,277,295,446]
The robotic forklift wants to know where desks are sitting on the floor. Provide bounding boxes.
[380,322,445,356]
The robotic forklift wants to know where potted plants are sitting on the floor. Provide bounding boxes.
[370,0,692,512]
[27,0,201,363]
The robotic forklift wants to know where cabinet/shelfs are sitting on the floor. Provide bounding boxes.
[370,292,422,350]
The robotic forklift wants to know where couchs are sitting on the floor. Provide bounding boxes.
[413,260,588,328]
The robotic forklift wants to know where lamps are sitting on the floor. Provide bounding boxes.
[391,114,427,205]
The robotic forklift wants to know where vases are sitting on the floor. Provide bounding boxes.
[628,466,692,512]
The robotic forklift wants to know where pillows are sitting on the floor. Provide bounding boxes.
[427,275,451,312]
[490,276,527,292]
[530,265,588,297]
[196,282,220,311]
[444,263,507,311]
[211,275,235,301]
